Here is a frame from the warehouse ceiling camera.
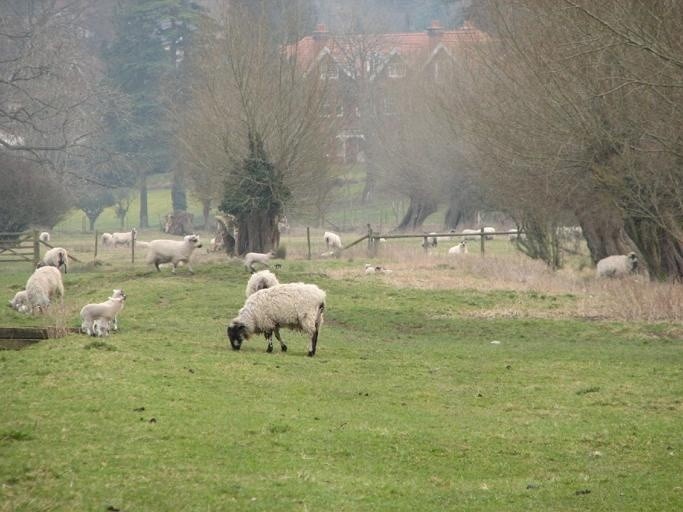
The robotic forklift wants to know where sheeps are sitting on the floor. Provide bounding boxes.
[101,227,139,247]
[422,226,528,255]
[225,279,328,357]
[144,233,201,275]
[39,230,51,243]
[79,288,128,337]
[323,230,343,254]
[596,251,638,277]
[7,247,69,318]
[363,263,394,275]
[244,250,281,300]
